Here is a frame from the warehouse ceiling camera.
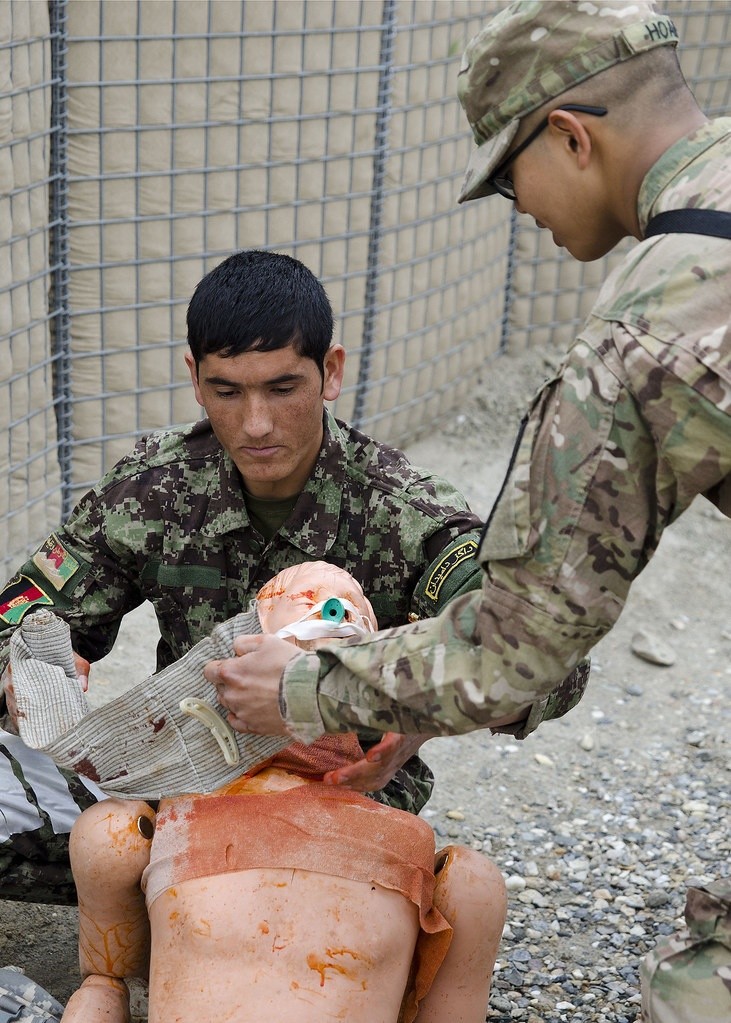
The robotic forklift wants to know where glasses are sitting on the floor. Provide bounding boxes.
[487,103,608,201]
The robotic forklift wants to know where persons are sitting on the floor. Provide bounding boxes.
[57,562,507,1023]
[0,251,588,907]
[200,0,731,743]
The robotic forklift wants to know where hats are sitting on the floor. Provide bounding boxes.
[457,1,680,204]
